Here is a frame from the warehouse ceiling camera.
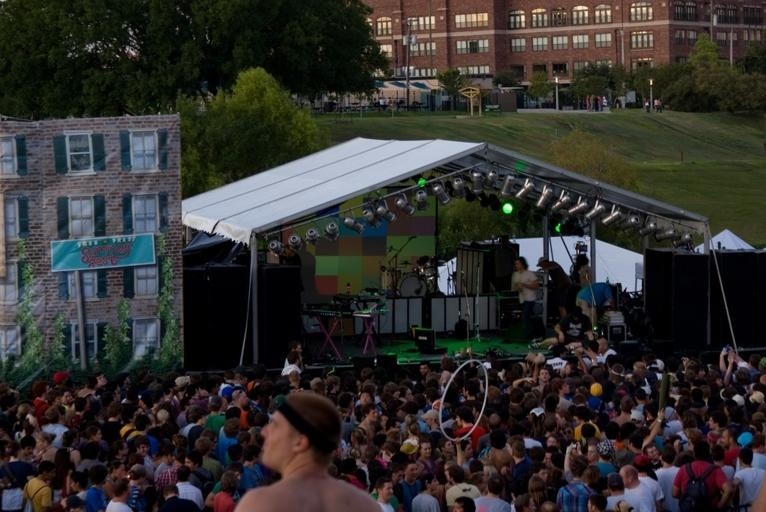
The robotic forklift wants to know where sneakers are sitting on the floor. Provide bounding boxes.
[528,343,542,350]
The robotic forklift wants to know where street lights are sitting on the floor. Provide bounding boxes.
[553,76,561,110]
[648,78,656,113]
[401,17,416,113]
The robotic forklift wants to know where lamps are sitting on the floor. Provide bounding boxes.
[262,162,694,258]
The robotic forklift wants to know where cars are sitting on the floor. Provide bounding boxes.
[602,96,609,107]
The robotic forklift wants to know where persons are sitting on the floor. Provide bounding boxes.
[586,94,665,114]
[2,345,766,512]
[493,233,633,345]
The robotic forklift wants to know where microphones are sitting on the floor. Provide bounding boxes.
[459,270,465,281]
[407,235,417,239]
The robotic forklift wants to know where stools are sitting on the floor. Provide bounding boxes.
[597,320,627,342]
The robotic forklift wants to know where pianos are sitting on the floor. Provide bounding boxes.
[303,308,376,319]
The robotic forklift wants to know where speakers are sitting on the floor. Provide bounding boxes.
[201,262,250,369]
[710,250,766,347]
[644,247,708,347]
[259,263,303,366]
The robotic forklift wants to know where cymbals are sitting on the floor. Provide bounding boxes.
[399,262,412,265]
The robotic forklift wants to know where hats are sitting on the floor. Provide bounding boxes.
[537,257,548,266]
[421,409,439,421]
[401,439,419,455]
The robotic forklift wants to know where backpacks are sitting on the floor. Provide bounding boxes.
[20,484,46,512]
[679,463,721,512]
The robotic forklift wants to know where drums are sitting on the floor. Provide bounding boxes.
[396,272,427,296]
[380,270,401,292]
[417,265,437,277]
